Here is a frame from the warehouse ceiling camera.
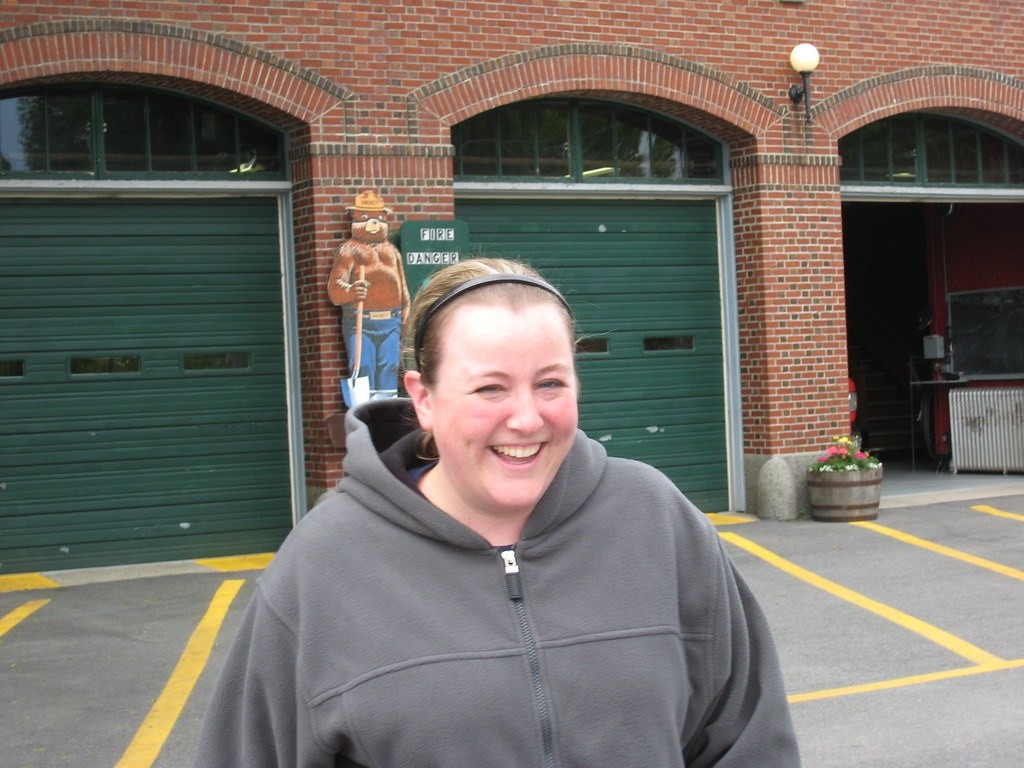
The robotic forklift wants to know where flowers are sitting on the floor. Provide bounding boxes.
[807,432,884,472]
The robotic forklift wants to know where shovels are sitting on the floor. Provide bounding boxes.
[339,264,370,411]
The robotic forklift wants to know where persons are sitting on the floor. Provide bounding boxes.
[195,259,800,768]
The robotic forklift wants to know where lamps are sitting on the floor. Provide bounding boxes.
[789,43,820,123]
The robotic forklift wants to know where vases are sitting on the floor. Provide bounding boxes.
[805,467,883,522]
